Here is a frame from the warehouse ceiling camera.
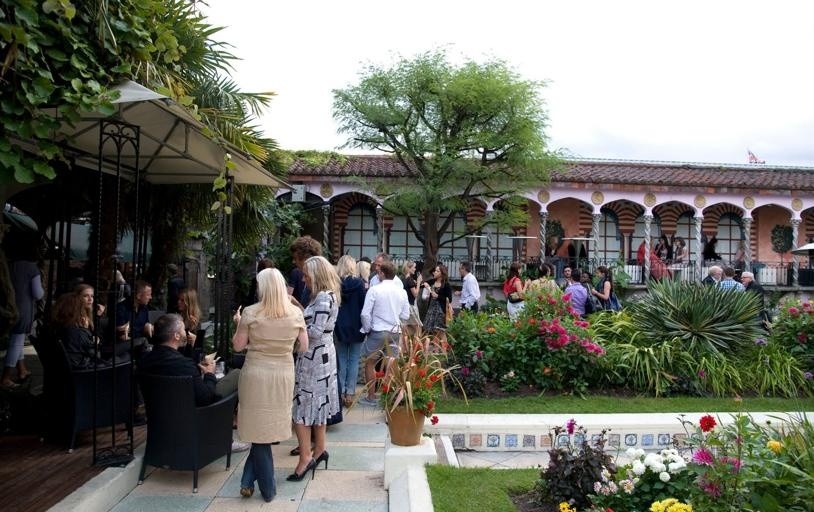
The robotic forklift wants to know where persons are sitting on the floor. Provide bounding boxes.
[6,237,452,500]
[638,236,764,308]
[505,236,616,319]
[460,260,481,315]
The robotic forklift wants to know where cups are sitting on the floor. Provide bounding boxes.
[215,362,224,376]
[147,345,153,351]
[416,272,420,277]
[200,353,207,377]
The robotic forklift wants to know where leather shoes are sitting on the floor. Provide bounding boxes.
[240,486,254,498]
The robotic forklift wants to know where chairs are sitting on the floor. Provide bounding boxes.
[131,372,238,495]
[27,335,46,365]
[35,327,135,454]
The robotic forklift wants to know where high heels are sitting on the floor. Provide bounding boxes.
[287,457,317,482]
[315,450,329,470]
[339,395,354,408]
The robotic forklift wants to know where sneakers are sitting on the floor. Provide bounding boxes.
[229,440,249,452]
[290,446,300,457]
[357,397,376,407]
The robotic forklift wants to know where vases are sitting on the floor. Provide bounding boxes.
[383,406,427,445]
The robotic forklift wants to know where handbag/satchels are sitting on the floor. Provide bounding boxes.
[585,283,602,314]
[445,298,454,329]
[506,278,526,303]
[601,279,623,311]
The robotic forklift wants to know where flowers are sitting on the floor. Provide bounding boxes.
[345,320,472,426]
